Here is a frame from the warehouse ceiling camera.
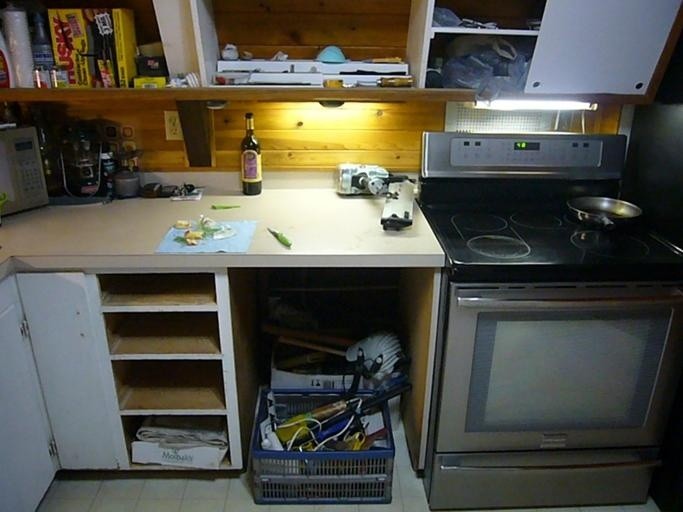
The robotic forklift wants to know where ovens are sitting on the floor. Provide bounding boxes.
[424,274,682,512]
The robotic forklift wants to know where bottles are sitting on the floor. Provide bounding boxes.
[96,33,116,88]
[239,111,262,196]
[32,12,54,68]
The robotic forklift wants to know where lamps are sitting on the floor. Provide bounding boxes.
[466,101,600,111]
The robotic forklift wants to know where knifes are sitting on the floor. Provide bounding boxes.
[266,224,292,248]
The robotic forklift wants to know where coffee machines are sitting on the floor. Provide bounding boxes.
[47,115,122,206]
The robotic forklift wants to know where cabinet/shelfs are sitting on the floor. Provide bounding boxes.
[0,217,58,510]
[0,0,683,101]
[8,186,445,471]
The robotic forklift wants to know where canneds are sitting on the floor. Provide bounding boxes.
[32,65,52,89]
[49,65,69,89]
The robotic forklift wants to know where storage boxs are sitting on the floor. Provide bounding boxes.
[251,386,396,505]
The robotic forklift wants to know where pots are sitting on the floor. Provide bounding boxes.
[563,196,644,229]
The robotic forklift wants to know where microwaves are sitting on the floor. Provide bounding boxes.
[0,125,51,222]
